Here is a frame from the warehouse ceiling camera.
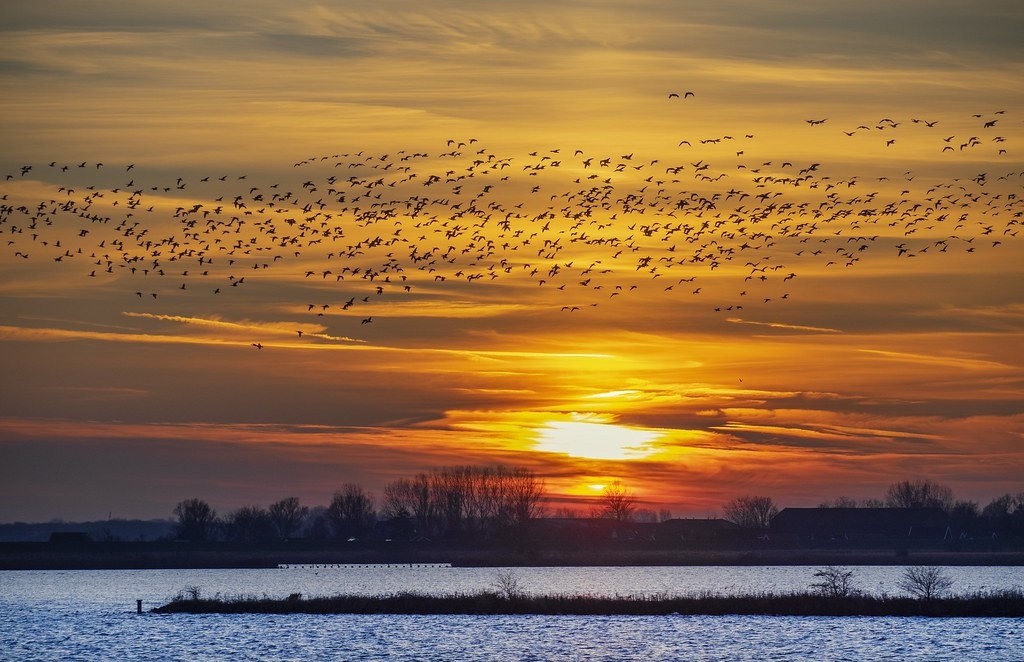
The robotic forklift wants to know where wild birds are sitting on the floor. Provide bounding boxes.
[668,93,679,100]
[739,378,743,383]
[684,92,694,99]
[0,110,1024,351]
